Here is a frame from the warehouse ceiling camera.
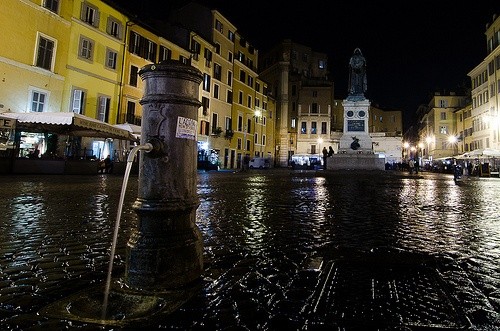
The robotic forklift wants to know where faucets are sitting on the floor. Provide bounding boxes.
[126,142,153,162]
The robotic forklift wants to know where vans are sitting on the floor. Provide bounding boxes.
[248,158,271,169]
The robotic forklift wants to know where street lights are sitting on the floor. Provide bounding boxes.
[425,136,433,155]
[449,136,456,155]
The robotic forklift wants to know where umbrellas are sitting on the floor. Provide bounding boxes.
[463,147,500,165]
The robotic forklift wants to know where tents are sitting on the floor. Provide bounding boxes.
[0,111,133,160]
[0,114,17,152]
[110,121,143,161]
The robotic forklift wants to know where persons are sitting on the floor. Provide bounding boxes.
[385,161,409,171]
[414,158,419,174]
[409,158,416,174]
[87,153,111,170]
[424,157,480,181]
[243,154,250,169]
[26,146,65,159]
[489,158,495,171]
[322,146,334,169]
